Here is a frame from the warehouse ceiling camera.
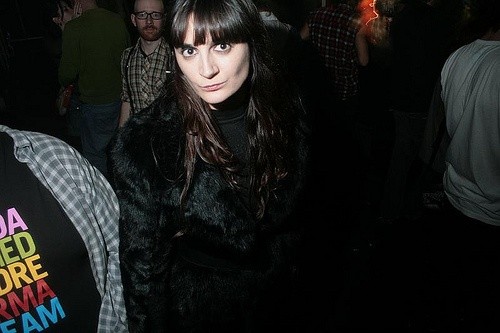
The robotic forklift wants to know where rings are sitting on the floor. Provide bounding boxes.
[77,12,80,14]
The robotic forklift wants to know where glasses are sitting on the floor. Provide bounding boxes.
[133,11,165,20]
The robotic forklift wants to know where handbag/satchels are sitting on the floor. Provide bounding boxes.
[56,85,73,116]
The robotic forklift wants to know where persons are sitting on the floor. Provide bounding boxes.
[118,0,176,128]
[420,2,500,333]
[300,0,377,214]
[0,125,129,333]
[59,0,133,180]
[369,0,477,250]
[107,0,343,333]
[50,0,83,155]
